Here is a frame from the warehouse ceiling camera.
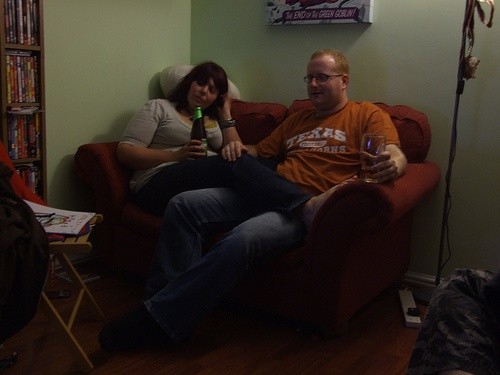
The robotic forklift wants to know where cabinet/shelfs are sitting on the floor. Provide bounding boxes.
[0,1,48,205]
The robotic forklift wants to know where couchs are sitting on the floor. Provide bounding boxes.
[69,98,445,339]
[405,267,500,375]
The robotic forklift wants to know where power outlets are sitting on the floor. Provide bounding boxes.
[398,287,425,329]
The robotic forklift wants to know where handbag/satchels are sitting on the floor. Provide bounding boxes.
[0,162,52,347]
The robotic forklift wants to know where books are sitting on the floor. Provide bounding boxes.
[4,0,42,197]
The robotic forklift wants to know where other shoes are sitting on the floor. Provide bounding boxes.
[97,306,171,354]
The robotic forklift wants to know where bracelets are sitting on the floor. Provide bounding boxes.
[218,120,237,129]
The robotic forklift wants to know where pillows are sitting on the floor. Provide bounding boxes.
[158,63,241,103]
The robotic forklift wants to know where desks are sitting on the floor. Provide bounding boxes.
[24,199,108,375]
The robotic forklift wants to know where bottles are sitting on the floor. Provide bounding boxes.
[191,105,208,160]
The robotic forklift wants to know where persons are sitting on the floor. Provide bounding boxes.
[0,142,48,209]
[117,61,358,230]
[0,167,47,349]
[97,49,408,352]
[405,268,500,375]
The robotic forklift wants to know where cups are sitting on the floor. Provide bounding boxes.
[359,132,387,184]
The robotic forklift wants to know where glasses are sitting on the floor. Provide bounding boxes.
[304,73,342,84]
